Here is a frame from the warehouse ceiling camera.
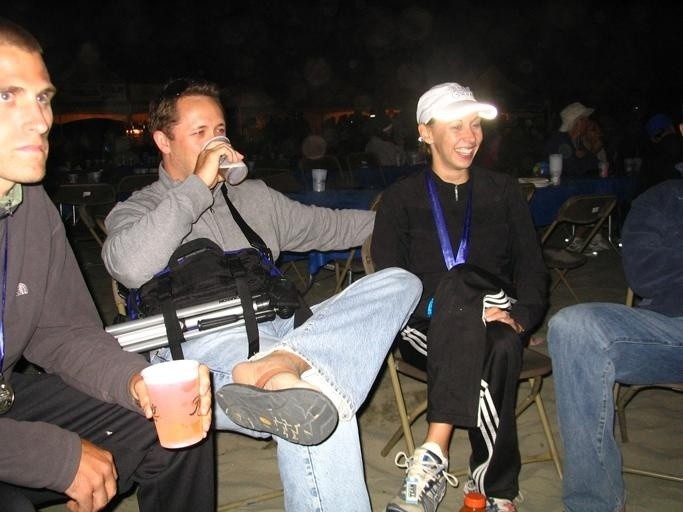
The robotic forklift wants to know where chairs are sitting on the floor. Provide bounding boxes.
[108,277,285,512]
[359,186,564,485]
[610,282,682,485]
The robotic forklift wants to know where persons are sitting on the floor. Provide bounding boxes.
[371,81,552,512]
[0,16,217,511]
[544,121,683,511]
[56,98,430,272]
[482,97,682,269]
[100,78,422,511]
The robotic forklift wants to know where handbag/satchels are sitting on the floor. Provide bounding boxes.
[132,239,274,319]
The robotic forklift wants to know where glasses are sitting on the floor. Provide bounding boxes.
[151,78,208,113]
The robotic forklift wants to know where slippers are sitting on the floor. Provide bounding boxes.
[213,364,338,448]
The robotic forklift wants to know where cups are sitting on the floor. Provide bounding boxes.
[311,169,327,193]
[201,135,250,187]
[596,162,609,178]
[548,153,562,187]
[138,358,204,451]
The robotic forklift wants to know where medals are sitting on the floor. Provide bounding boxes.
[0,382,15,417]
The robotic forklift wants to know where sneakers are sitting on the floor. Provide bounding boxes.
[387,445,459,511]
[463,480,525,512]
[589,241,604,251]
[566,240,584,251]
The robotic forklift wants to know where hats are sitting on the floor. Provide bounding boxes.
[558,102,594,134]
[646,113,672,137]
[416,83,497,127]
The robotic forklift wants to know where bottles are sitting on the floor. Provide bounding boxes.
[459,491,492,512]
